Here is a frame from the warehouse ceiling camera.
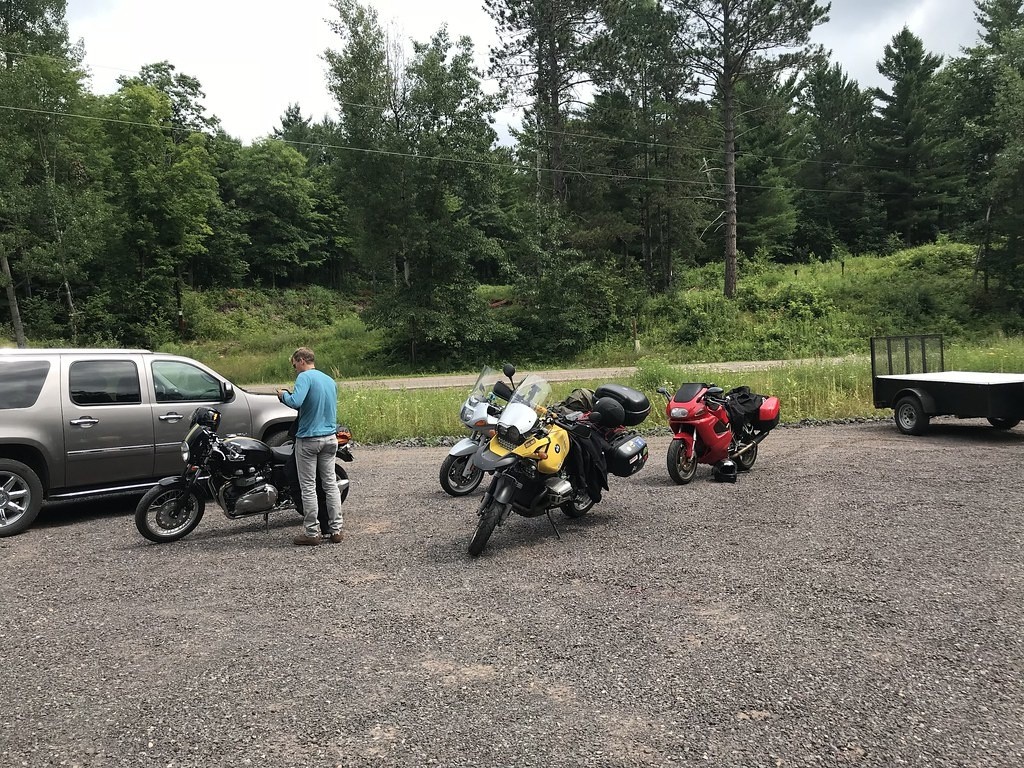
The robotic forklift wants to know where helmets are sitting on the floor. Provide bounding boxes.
[593,396,625,429]
[189,406,221,432]
[713,458,738,482]
[568,389,592,411]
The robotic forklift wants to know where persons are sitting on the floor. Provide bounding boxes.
[275,347,344,545]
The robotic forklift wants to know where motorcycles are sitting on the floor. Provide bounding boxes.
[134,421,354,543]
[655,383,780,485]
[467,362,652,556]
[439,364,596,497]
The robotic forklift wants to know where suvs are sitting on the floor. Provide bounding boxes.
[0,347,300,538]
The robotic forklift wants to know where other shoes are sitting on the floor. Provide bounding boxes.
[330,535,343,543]
[293,534,320,545]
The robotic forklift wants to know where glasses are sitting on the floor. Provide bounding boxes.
[293,358,304,368]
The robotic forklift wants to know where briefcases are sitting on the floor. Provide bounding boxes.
[606,430,649,477]
